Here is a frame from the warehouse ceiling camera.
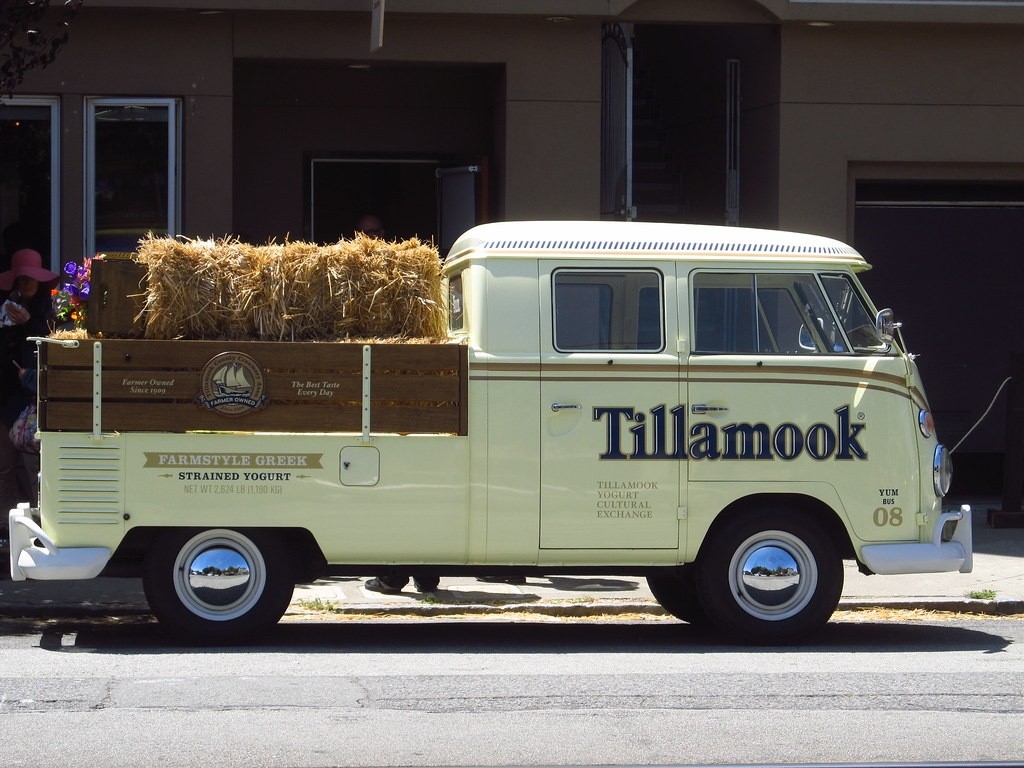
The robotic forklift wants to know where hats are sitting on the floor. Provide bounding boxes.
[0,249,63,290]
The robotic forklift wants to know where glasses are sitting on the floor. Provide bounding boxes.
[359,227,383,236]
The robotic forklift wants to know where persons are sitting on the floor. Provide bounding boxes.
[0,248,58,433]
[11,367,40,505]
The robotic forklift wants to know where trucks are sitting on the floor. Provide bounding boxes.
[28,222,976,647]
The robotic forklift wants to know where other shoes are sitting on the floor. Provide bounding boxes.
[416,586,437,593]
[365,577,401,595]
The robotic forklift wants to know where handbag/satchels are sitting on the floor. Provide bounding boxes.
[8,392,40,454]
